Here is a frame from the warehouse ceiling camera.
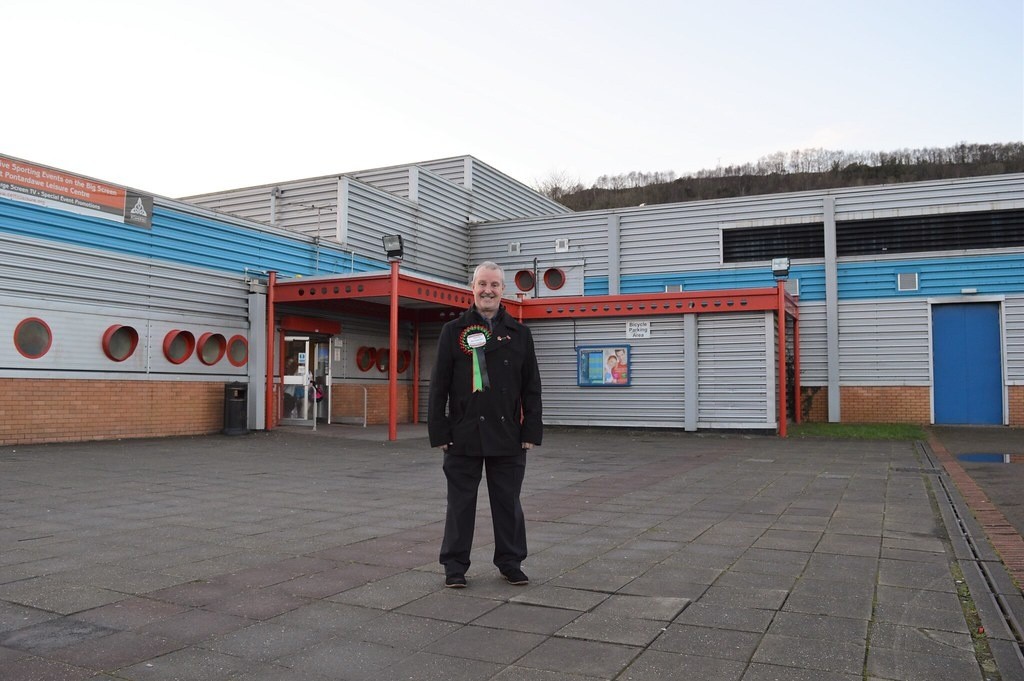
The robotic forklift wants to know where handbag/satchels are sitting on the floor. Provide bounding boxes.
[308,381,323,402]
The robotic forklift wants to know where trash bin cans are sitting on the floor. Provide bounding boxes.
[221,381,250,436]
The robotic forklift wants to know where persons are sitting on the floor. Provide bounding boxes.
[427,261,546,587]
[604,349,627,384]
[292,363,314,419]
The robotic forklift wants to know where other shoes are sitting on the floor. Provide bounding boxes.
[445,574,467,587]
[498,567,529,584]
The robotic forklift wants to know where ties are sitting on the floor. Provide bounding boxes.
[485,319,492,335]
